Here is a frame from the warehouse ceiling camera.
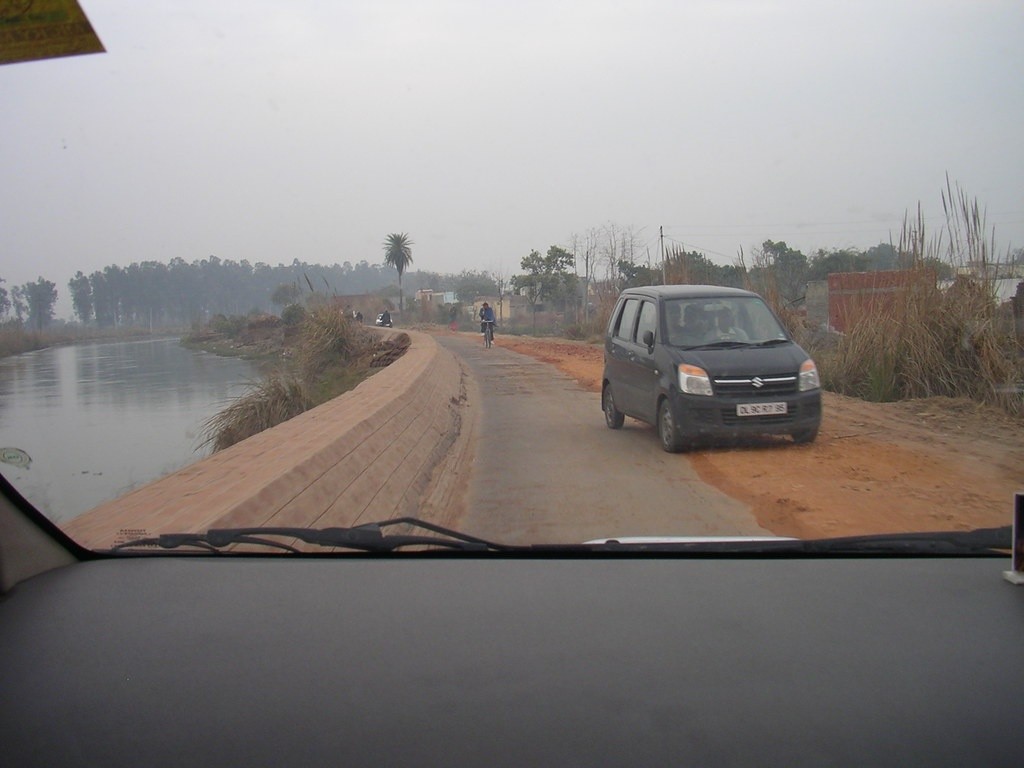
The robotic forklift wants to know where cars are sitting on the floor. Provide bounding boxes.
[376,313,393,326]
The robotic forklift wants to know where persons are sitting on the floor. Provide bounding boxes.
[381,310,390,324]
[667,302,748,342]
[479,303,496,345]
[356,312,363,323]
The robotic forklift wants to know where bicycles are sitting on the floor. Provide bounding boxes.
[479,319,494,350]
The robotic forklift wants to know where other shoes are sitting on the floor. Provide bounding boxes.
[481,333,485,336]
[491,341,494,345]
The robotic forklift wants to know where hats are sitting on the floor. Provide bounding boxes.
[481,303,488,306]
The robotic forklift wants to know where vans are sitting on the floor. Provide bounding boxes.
[600,284,824,455]
